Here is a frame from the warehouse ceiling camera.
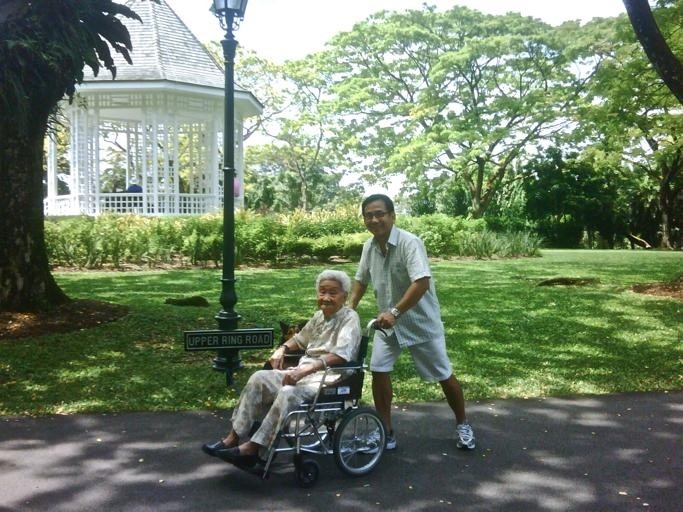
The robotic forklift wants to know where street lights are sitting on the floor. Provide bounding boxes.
[212,0,249,372]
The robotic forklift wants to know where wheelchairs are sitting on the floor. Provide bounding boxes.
[203,319,388,487]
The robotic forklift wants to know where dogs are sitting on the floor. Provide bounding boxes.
[261,320,309,369]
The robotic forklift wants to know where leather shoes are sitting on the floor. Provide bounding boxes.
[201,438,238,456]
[214,446,257,467]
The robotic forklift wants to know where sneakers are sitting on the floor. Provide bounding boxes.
[455,424,476,450]
[360,429,396,454]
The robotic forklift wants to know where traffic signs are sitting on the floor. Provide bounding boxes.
[185,325,274,350]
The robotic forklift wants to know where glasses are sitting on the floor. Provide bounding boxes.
[363,211,390,220]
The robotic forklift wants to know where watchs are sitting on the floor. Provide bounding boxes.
[389,306,402,319]
[277,344,290,350]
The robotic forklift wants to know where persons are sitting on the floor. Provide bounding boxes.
[202,269,362,469]
[342,193,476,450]
